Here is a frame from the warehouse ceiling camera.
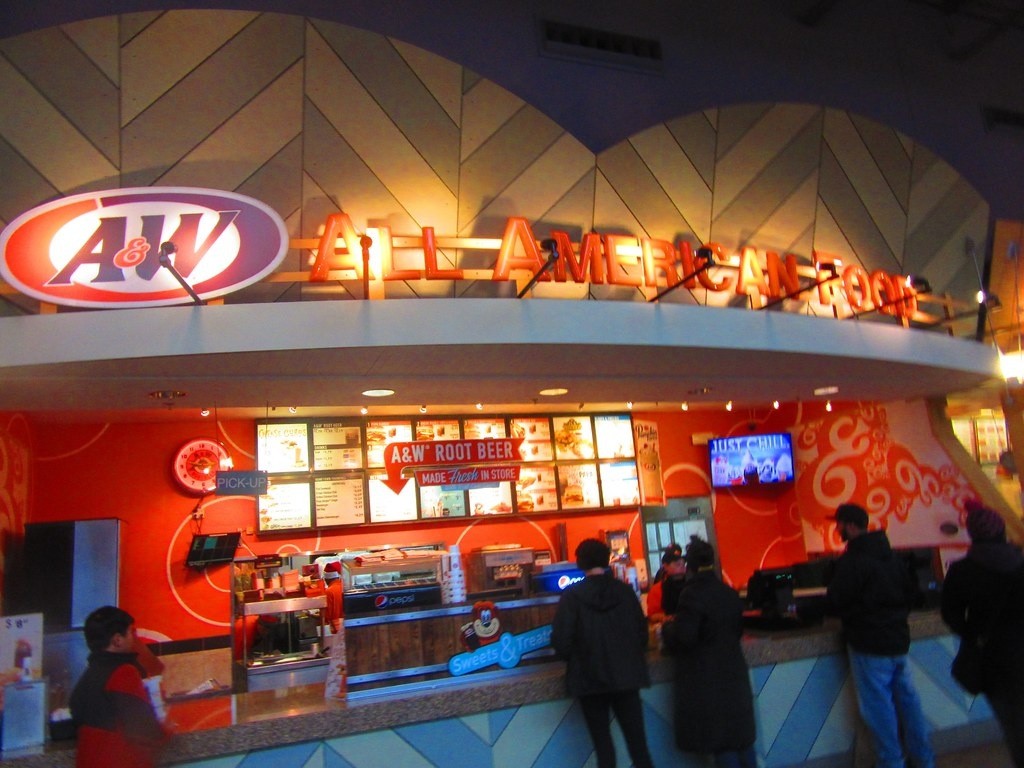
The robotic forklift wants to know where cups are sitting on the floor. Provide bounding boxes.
[459,622,480,650]
[639,450,663,506]
[730,477,744,486]
[141,676,168,721]
[714,463,728,484]
[324,625,330,636]
[315,625,322,636]
[311,643,320,655]
[743,467,759,485]
[777,467,789,482]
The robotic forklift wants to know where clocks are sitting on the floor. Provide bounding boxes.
[170,436,234,498]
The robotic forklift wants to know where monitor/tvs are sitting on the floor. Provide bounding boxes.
[187,532,241,566]
[708,433,794,488]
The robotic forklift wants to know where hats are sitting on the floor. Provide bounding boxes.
[837,506,867,523]
[966,508,1005,543]
[684,541,714,566]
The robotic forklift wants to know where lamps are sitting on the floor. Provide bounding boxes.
[917,289,1004,330]
[954,238,1018,406]
[648,246,717,305]
[848,275,932,318]
[357,234,374,301]
[755,261,839,311]
[159,241,206,307]
[516,239,560,300]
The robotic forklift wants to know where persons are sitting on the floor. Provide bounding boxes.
[551,538,653,768]
[791,504,936,768]
[72,606,170,768]
[655,537,758,768]
[646,552,686,624]
[939,501,1023,768]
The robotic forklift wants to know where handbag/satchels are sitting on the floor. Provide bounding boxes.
[951,613,987,697]
